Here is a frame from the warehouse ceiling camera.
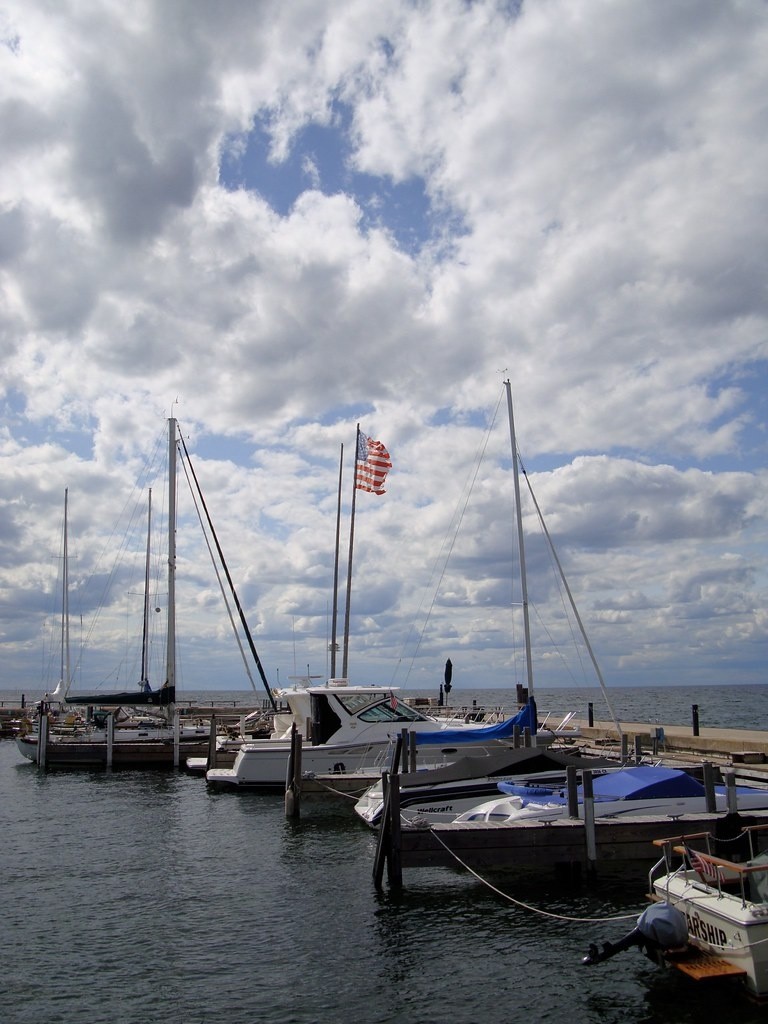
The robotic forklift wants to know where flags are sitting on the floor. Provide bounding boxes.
[356,431,391,495]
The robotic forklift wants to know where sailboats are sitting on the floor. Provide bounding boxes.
[10,366,668,835]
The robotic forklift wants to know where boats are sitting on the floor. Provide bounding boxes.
[375,727,768,872]
[581,822,767,1013]
